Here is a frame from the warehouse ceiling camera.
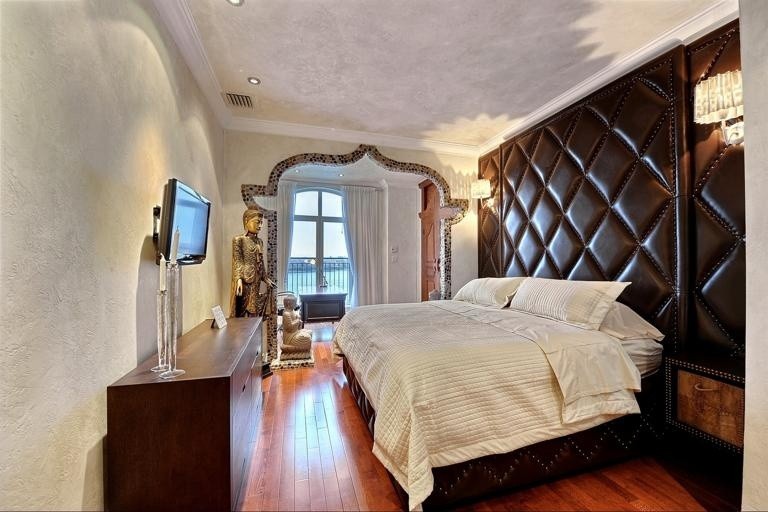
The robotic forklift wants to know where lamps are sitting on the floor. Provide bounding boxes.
[692,70,746,147]
[471,180,494,209]
[309,256,328,286]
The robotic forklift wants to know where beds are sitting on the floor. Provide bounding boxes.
[332,286,746,512]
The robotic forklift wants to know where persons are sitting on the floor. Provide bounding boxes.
[283,295,313,346]
[230,209,278,318]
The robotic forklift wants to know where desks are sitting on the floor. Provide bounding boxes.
[300,284,350,330]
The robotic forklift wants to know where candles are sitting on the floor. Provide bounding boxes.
[160,253,167,290]
[169,226,180,264]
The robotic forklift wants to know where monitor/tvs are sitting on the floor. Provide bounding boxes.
[157,178,213,266]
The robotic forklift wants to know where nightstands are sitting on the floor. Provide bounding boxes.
[664,356,746,455]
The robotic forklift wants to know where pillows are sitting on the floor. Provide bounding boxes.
[508,277,632,332]
[452,278,525,308]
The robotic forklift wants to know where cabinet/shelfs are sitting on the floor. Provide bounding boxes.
[106,316,263,512]
